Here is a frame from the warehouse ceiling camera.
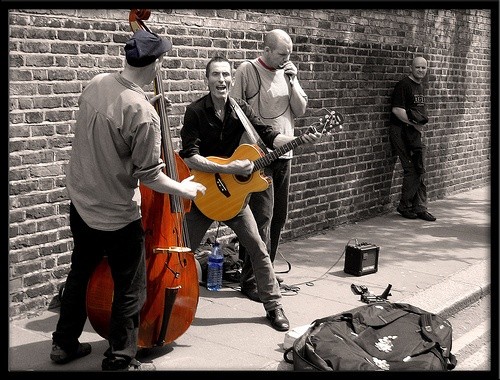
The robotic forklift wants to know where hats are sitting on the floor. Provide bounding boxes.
[124,30,172,67]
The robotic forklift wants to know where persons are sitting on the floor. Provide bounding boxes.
[48,30,207,372]
[388,57,437,221]
[176,56,289,331]
[229,28,309,283]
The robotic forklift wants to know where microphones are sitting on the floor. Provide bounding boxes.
[283,63,293,84]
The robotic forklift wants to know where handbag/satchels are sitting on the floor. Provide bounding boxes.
[284,299,457,371]
[222,248,244,283]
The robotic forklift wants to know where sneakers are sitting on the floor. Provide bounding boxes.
[397,205,417,219]
[411,207,436,221]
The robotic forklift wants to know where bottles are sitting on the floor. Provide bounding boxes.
[207,241,224,291]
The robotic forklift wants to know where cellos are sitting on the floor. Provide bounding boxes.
[85,9,200,351]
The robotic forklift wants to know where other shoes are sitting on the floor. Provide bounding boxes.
[241,285,262,302]
[101,358,155,372]
[50,338,92,360]
[280,285,286,293]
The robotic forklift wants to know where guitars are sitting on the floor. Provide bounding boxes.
[191,110,344,222]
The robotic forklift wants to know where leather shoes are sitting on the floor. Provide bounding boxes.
[266,308,289,330]
